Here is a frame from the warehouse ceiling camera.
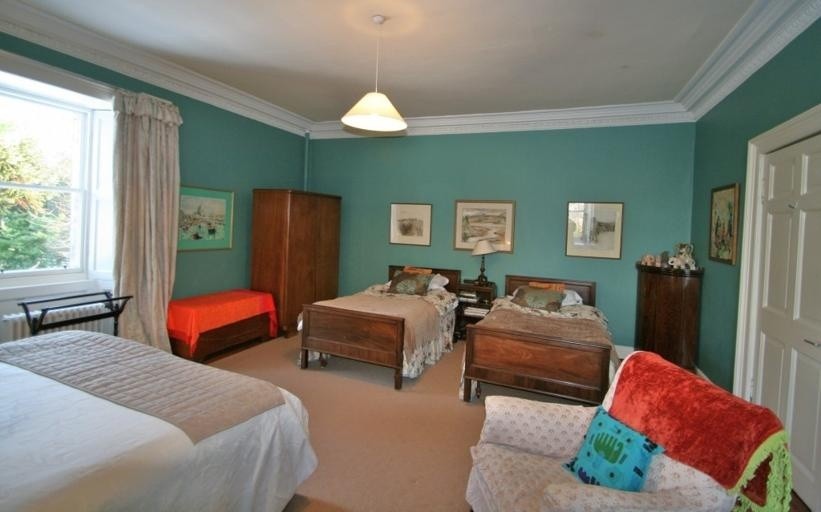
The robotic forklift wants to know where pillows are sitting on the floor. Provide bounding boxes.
[562,403,666,493]
[389,271,433,297]
[401,265,431,275]
[529,281,568,293]
[511,284,567,313]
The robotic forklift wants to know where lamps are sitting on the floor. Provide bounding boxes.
[340,14,411,134]
[469,239,496,282]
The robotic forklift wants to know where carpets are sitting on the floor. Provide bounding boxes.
[280,492,354,511]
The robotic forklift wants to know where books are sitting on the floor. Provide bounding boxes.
[459,290,490,319]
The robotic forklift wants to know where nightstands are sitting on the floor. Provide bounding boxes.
[455,282,496,341]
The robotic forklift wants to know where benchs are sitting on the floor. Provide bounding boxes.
[168,288,278,359]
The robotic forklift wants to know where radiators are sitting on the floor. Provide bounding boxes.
[0,302,115,343]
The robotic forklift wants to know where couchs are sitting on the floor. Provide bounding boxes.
[462,351,794,512]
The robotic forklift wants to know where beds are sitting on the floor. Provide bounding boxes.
[464,272,609,407]
[0,329,319,512]
[300,263,461,391]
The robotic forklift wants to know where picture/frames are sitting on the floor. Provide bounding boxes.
[707,180,740,267]
[178,186,236,255]
[388,200,432,247]
[453,199,517,254]
[565,201,624,261]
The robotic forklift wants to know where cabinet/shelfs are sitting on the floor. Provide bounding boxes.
[634,264,700,374]
[251,190,341,337]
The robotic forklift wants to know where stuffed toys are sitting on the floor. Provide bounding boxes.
[640,242,696,269]
